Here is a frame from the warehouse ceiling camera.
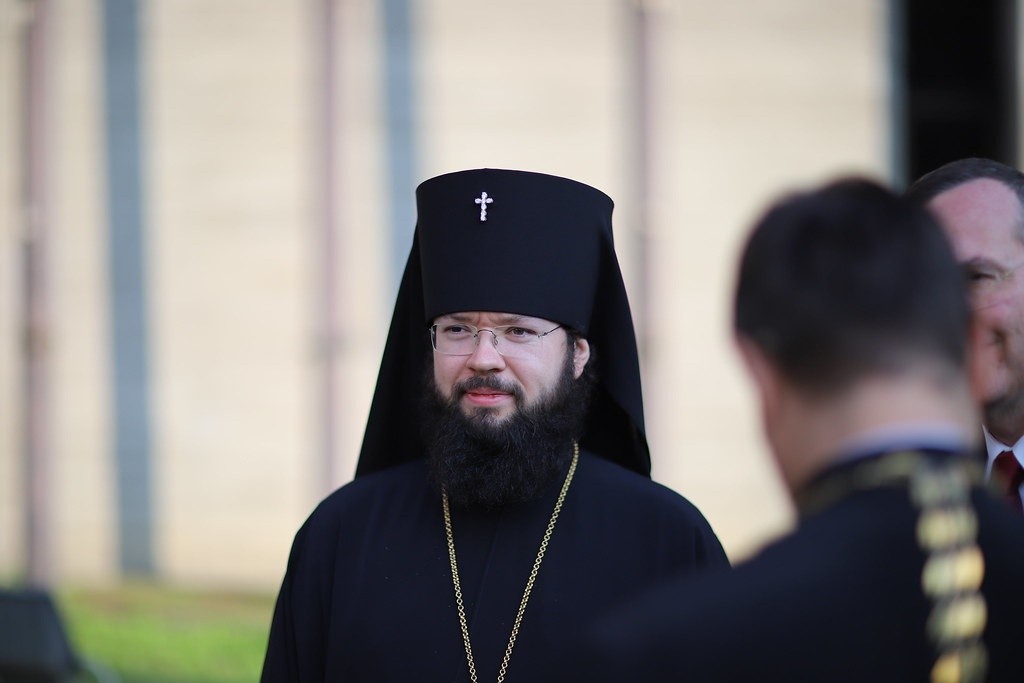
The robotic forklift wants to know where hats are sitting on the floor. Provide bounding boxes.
[354,167,651,478]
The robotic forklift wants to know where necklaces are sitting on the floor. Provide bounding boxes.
[441,438,578,683]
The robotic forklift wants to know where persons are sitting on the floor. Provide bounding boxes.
[259,167,732,683]
[593,158,1024,683]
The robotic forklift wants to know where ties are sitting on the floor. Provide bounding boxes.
[989,451,1024,519]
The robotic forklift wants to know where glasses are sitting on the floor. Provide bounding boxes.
[428,322,561,359]
[961,261,1024,313]
